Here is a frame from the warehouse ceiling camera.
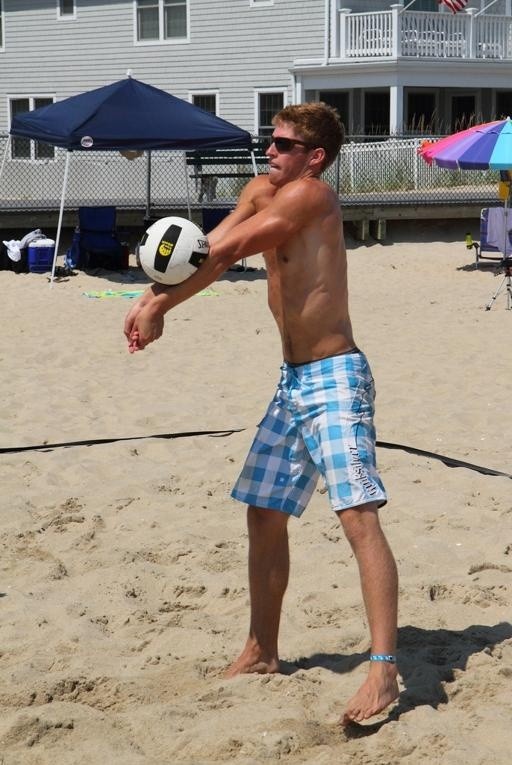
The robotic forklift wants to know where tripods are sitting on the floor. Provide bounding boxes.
[486,267,512,309]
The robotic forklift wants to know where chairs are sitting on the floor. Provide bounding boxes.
[473,208,512,269]
[78,205,124,275]
[360,29,503,55]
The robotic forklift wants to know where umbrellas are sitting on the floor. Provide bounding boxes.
[418,116,512,259]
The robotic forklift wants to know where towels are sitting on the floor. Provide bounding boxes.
[486,207,512,255]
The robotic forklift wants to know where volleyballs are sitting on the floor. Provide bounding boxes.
[138,217,211,285]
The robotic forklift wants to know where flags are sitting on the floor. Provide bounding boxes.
[437,0,469,15]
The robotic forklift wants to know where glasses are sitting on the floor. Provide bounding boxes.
[269,134,318,153]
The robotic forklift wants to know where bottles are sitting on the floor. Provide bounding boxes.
[465,232,472,249]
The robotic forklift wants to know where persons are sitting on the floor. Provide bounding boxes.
[123,104,399,722]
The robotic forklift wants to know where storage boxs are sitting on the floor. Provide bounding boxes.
[27,238,56,273]
